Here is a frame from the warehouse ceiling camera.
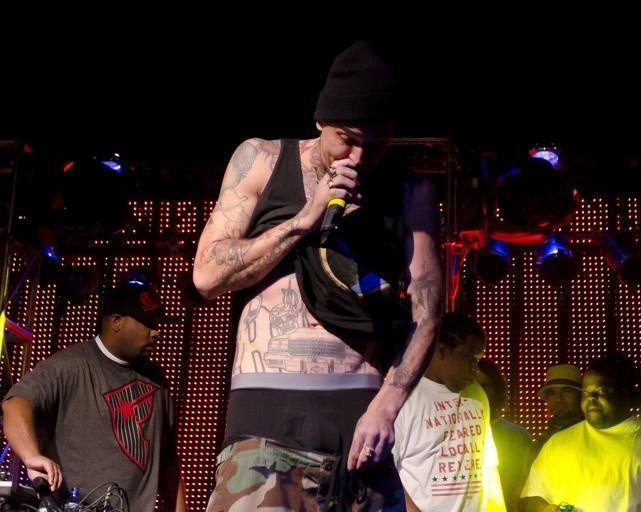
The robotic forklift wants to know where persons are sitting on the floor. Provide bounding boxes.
[510,363,586,511]
[473,357,533,512]
[512,351,641,511]
[193,38,445,512]
[391,310,508,512]
[0,277,189,512]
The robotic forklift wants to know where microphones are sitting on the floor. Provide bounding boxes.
[321,159,358,238]
[32,477,64,503]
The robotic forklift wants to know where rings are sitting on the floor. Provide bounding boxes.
[361,445,374,457]
[327,179,334,188]
[328,167,337,178]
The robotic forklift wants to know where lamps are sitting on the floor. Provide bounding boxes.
[472,141,641,288]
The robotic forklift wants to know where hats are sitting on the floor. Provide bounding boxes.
[538,365,583,400]
[99,281,180,326]
[315,30,396,121]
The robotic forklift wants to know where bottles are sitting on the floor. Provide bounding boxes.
[65,486,83,511]
